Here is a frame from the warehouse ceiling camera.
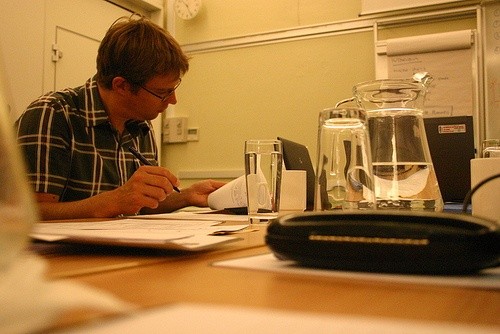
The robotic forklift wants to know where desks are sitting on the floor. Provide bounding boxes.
[34,209,500,334]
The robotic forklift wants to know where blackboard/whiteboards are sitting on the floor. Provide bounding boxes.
[482,3,500,148]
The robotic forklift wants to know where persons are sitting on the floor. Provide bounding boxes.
[12,13,228,221]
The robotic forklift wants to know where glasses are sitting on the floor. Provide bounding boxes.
[131,77,182,102]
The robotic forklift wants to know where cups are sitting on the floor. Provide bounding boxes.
[478,138,500,158]
[312,107,377,210]
[245,139,283,228]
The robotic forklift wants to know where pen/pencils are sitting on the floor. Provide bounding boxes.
[126,143,181,193]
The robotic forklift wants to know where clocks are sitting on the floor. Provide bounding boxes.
[175,0,202,20]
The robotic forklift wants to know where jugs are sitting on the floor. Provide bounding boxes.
[336,70,445,212]
